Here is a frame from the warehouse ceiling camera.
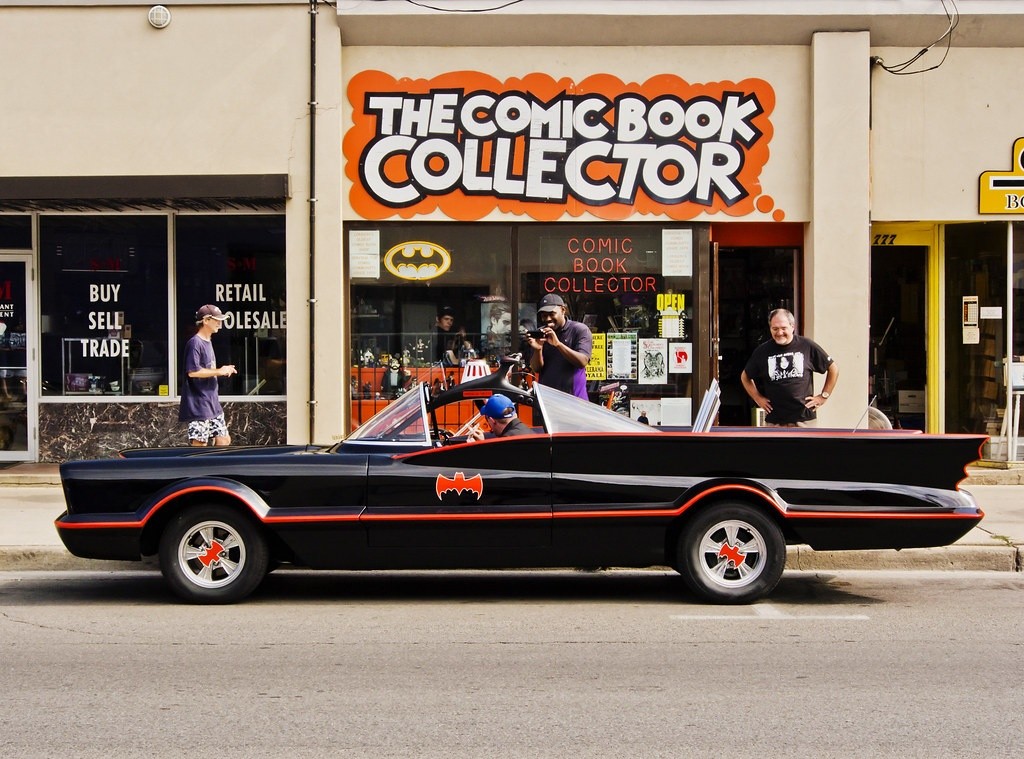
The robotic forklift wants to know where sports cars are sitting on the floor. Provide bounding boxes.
[53,353,991,607]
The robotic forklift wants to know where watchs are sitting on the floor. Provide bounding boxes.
[821,391,830,400]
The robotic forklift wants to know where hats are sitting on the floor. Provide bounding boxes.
[537,293,565,314]
[437,304,456,315]
[196,304,230,321]
[480,393,515,419]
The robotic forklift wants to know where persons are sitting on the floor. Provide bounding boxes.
[485,301,536,362]
[465,394,539,443]
[741,309,839,428]
[528,294,593,402]
[422,306,480,367]
[177,305,238,448]
[349,338,458,400]
[259,327,286,394]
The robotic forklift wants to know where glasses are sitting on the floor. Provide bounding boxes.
[500,318,512,326]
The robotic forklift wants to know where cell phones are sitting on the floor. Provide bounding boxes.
[526,330,546,339]
